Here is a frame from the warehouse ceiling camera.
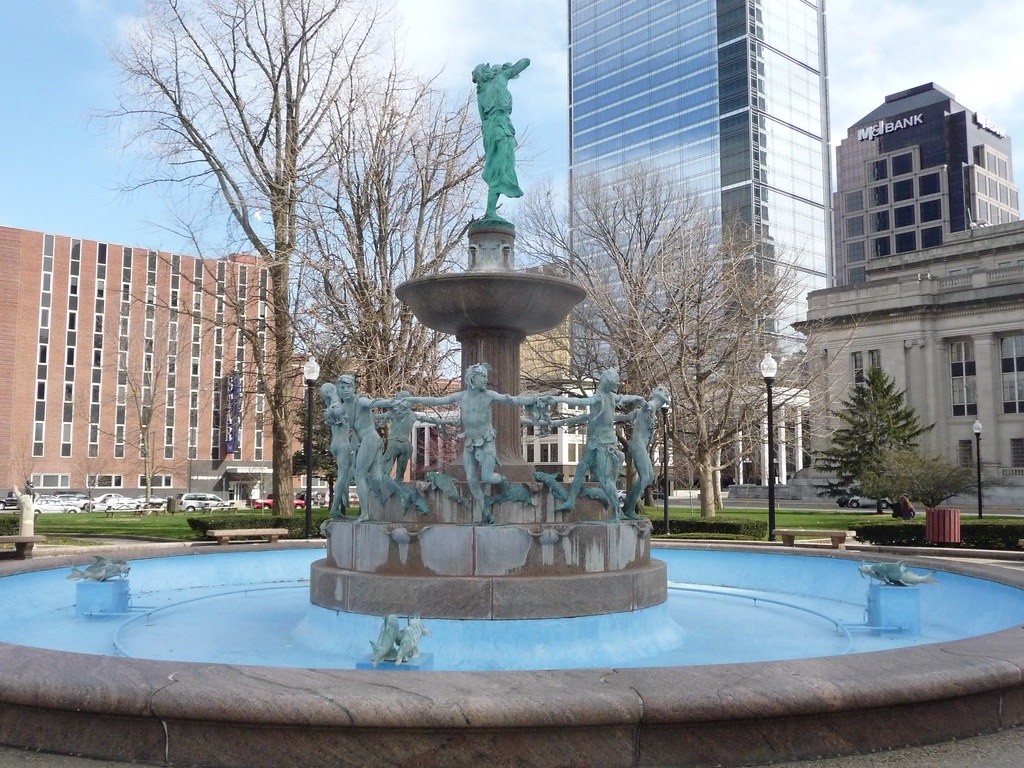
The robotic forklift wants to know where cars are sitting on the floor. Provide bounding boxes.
[617,489,627,502]
[848,493,893,510]
[652,490,665,500]
[0,489,359,513]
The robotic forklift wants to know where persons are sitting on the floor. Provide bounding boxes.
[318,363,671,524]
[471,57,531,220]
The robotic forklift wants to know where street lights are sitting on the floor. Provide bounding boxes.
[972,419,982,519]
[660,394,673,536]
[302,356,321,539]
[759,352,777,542]
